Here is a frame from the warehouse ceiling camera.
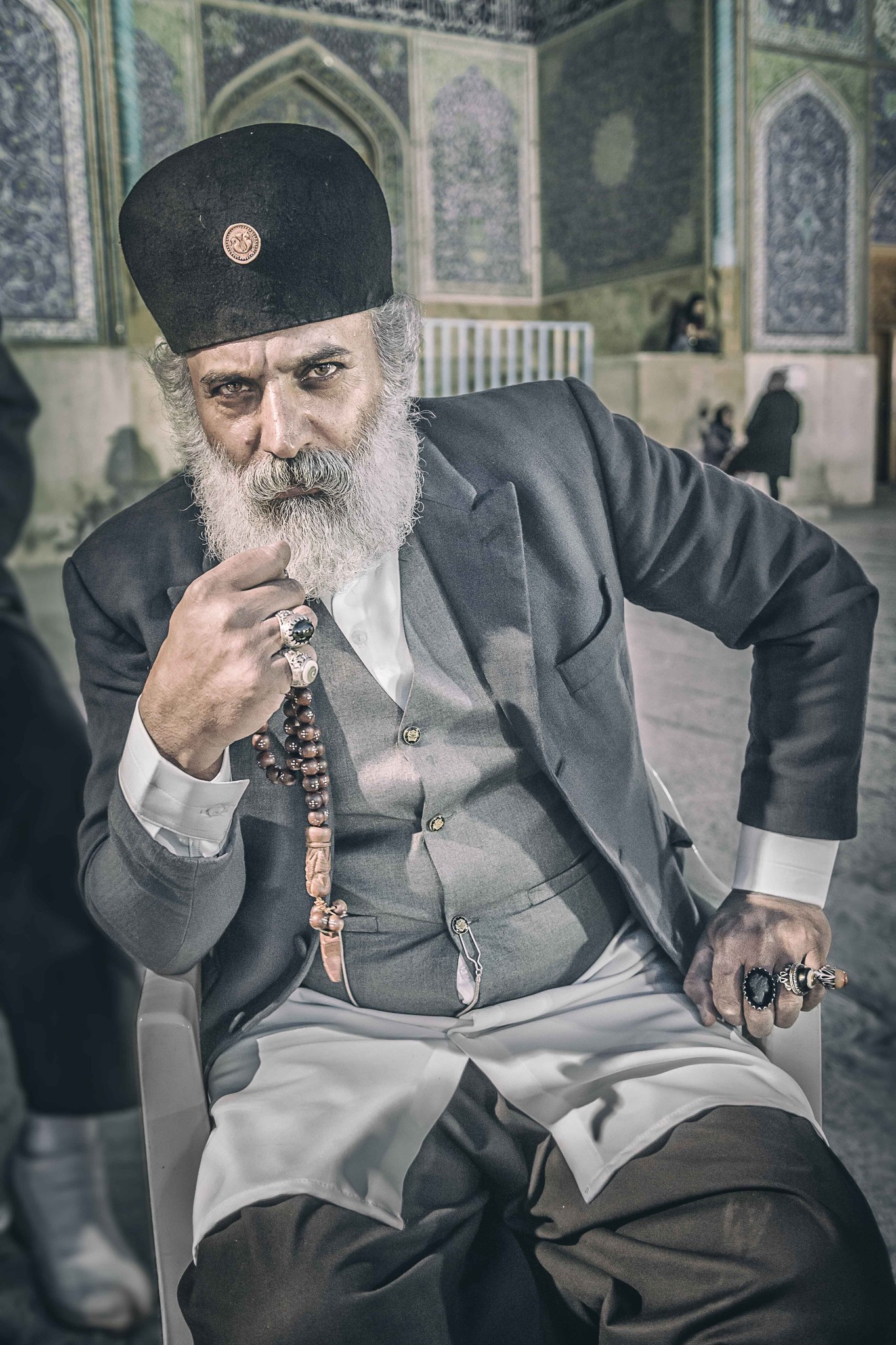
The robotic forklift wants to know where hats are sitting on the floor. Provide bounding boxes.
[118,123,393,354]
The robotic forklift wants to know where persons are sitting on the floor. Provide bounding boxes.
[0,309,152,1332]
[705,405,734,468]
[60,121,896,1345]
[728,370,800,501]
[669,293,720,355]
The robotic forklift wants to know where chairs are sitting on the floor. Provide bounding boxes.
[135,767,824,1344]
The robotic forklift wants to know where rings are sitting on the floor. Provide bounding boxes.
[773,963,816,995]
[814,964,848,991]
[744,967,776,1011]
[275,611,316,648]
[277,651,318,688]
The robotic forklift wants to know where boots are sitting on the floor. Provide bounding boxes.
[10,1125,159,1333]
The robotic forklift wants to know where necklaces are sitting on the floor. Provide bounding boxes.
[253,685,348,982]
